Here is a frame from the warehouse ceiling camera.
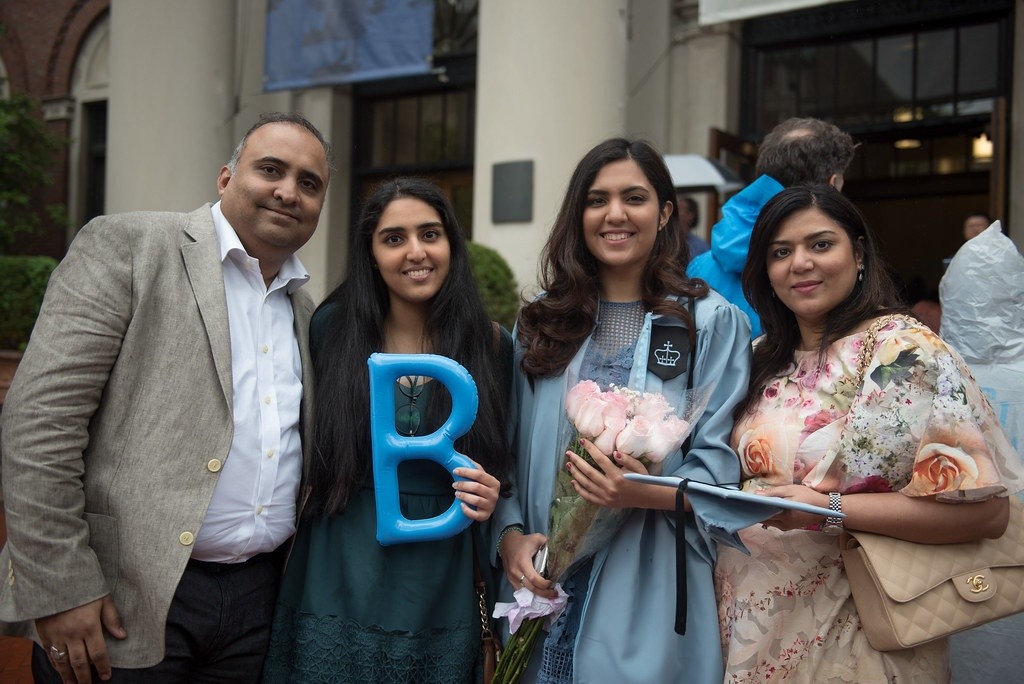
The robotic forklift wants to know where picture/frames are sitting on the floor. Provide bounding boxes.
[708,127,753,186]
[673,186,719,248]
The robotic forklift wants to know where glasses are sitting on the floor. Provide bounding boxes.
[395,374,425,436]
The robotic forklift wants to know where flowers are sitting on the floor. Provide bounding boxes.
[490,378,691,683]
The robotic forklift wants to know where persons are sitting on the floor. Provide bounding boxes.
[491,116,1024,684]
[0,111,336,684]
[264,175,514,684]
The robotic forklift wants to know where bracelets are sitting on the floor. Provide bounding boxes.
[495,526,524,558]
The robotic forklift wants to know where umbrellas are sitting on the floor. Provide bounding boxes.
[662,153,746,197]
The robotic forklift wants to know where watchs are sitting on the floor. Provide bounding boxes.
[821,493,843,537]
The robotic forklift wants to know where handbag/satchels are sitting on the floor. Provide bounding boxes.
[836,312,1024,652]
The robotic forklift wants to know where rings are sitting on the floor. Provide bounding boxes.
[762,523,768,529]
[519,575,525,584]
[50,645,67,660]
